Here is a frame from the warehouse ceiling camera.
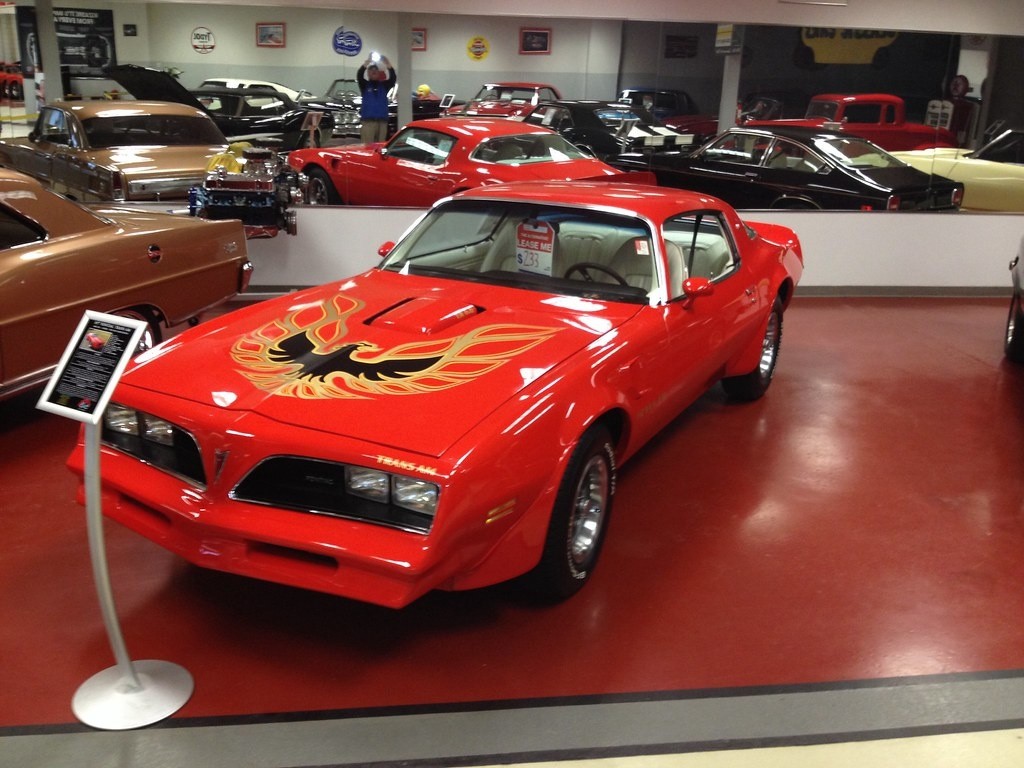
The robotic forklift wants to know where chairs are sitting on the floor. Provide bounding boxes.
[481,219,565,280]
[495,144,523,160]
[602,235,690,301]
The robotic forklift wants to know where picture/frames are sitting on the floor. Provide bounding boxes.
[518,25,553,54]
[256,22,286,47]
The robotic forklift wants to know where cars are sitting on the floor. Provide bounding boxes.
[740,94,959,153]
[527,99,701,165]
[1,169,253,401]
[66,180,803,612]
[882,119,1024,212]
[188,88,335,151]
[300,77,465,143]
[662,92,783,137]
[290,118,658,209]
[194,77,315,117]
[1,97,231,214]
[439,83,564,126]
[0,62,24,101]
[599,89,697,120]
[1003,236,1024,365]
[609,126,965,212]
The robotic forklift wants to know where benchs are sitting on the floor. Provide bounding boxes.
[554,219,729,282]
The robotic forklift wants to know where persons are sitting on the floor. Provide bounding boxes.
[357,52,397,143]
